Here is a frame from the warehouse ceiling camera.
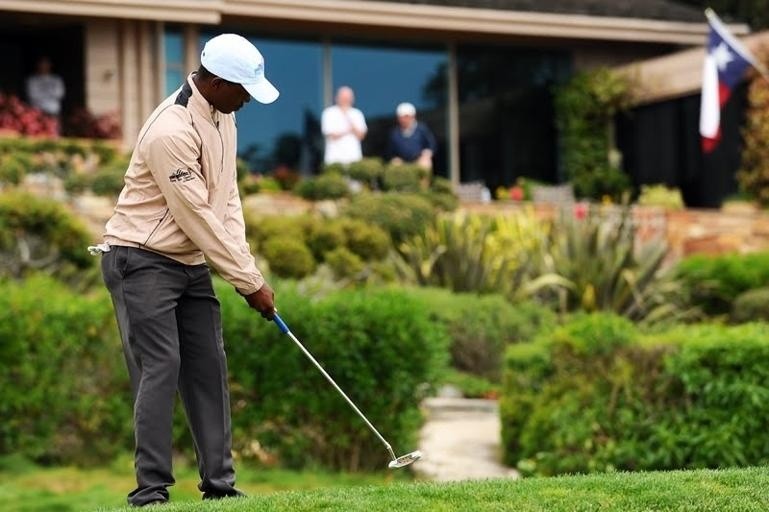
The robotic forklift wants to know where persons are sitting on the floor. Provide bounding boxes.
[390,101,435,186]
[104,30,280,506]
[24,54,65,138]
[321,84,368,168]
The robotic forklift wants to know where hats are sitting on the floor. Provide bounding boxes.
[396,102,416,117]
[200,33,282,107]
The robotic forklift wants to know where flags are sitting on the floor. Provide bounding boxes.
[698,15,756,154]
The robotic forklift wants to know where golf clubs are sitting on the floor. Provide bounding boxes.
[275,314,421,468]
